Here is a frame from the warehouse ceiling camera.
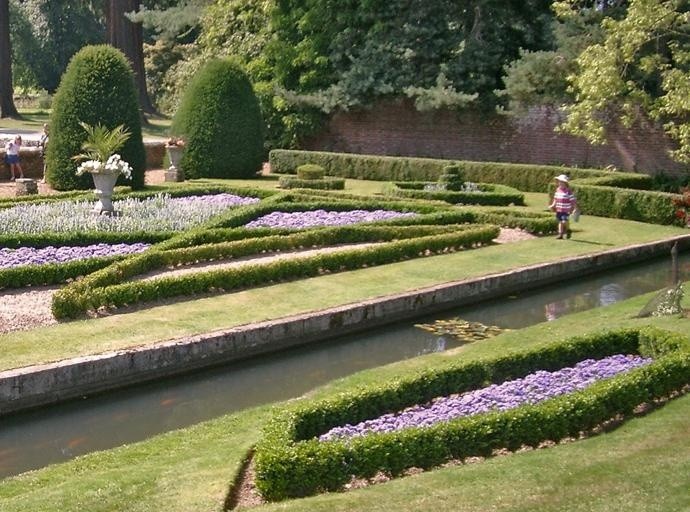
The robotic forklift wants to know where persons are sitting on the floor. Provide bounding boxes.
[39,122,50,184]
[547,174,578,240]
[4,134,25,182]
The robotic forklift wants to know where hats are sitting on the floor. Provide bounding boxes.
[554,174,568,184]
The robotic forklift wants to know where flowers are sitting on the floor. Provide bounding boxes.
[165,136,185,147]
[71,121,133,181]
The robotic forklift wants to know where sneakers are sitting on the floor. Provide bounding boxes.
[556,229,572,239]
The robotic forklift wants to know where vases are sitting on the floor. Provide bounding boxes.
[91,173,119,216]
[164,147,185,182]
